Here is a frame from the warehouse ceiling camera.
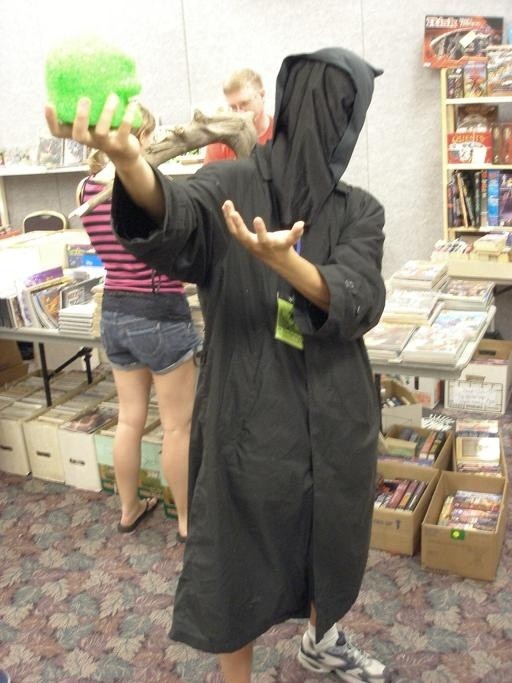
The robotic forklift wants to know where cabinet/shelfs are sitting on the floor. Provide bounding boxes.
[442,89,512,287]
[370,338,510,582]
[0,363,174,509]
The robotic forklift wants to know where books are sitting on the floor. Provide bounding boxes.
[2,227,204,337]
[363,259,506,531]
[0,362,167,499]
[435,45,512,293]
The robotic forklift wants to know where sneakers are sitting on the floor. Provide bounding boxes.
[295,627,392,683]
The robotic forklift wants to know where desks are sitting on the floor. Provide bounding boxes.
[1,303,498,434]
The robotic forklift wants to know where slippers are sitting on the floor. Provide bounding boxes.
[117,494,160,533]
[174,530,189,542]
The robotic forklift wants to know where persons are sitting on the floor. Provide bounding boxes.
[42,40,398,681]
[76,96,205,545]
[196,65,276,165]
